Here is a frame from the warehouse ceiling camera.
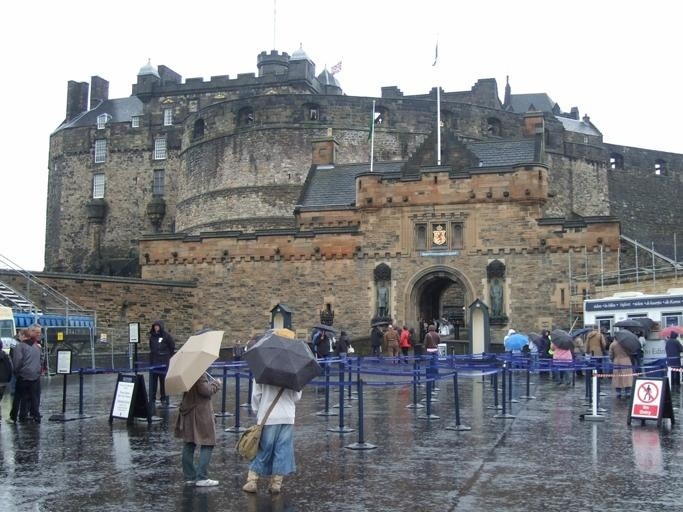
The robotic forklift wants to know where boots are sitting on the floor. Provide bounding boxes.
[242,470,259,492]
[268,475,283,493]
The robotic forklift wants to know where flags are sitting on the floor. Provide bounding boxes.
[367,110,374,142]
[330,60,341,76]
[431,36,438,68]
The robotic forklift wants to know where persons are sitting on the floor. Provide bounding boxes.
[369,317,456,365]
[311,324,351,371]
[149,321,170,409]
[174,372,222,488]
[232,335,257,372]
[504,326,683,400]
[243,377,302,493]
[0,322,45,423]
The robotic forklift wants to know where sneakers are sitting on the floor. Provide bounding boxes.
[185,480,196,487]
[196,479,219,487]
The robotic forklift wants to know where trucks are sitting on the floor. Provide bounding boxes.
[581,288,681,381]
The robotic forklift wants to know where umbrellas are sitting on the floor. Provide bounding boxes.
[243,328,324,393]
[633,317,656,329]
[163,329,226,397]
[612,319,642,329]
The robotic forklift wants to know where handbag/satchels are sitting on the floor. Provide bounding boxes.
[408,336,417,344]
[347,344,354,353]
[235,425,262,461]
